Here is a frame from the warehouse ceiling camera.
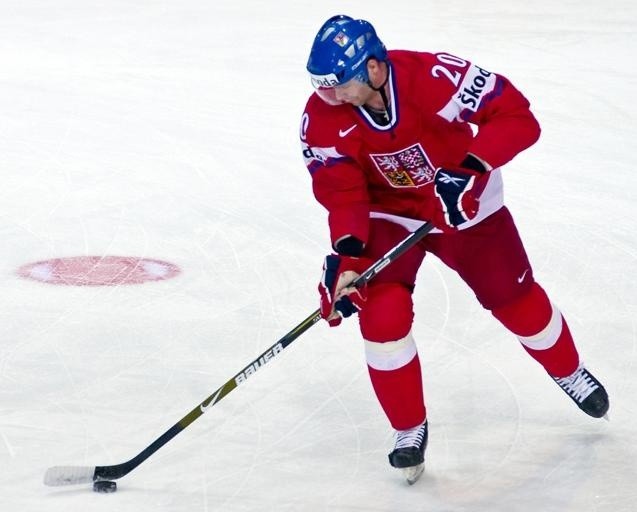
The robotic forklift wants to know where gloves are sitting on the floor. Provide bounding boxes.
[433,162,481,225]
[317,253,373,329]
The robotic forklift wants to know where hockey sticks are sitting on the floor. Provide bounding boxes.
[44,221,433,485]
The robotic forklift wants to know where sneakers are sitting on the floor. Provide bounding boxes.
[548,360,611,420]
[387,418,430,469]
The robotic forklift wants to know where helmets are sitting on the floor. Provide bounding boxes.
[306,14,388,88]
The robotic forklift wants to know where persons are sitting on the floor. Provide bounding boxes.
[300,14,609,468]
